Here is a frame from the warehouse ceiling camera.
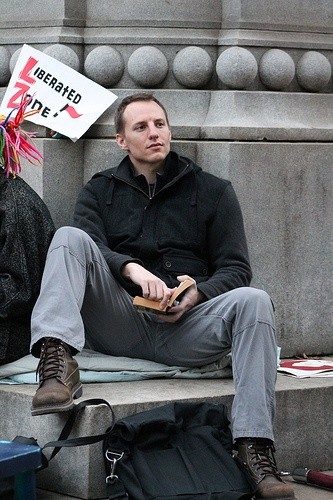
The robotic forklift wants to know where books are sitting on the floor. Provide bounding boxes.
[132,279,194,312]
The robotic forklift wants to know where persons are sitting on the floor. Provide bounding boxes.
[30,93,296,500]
